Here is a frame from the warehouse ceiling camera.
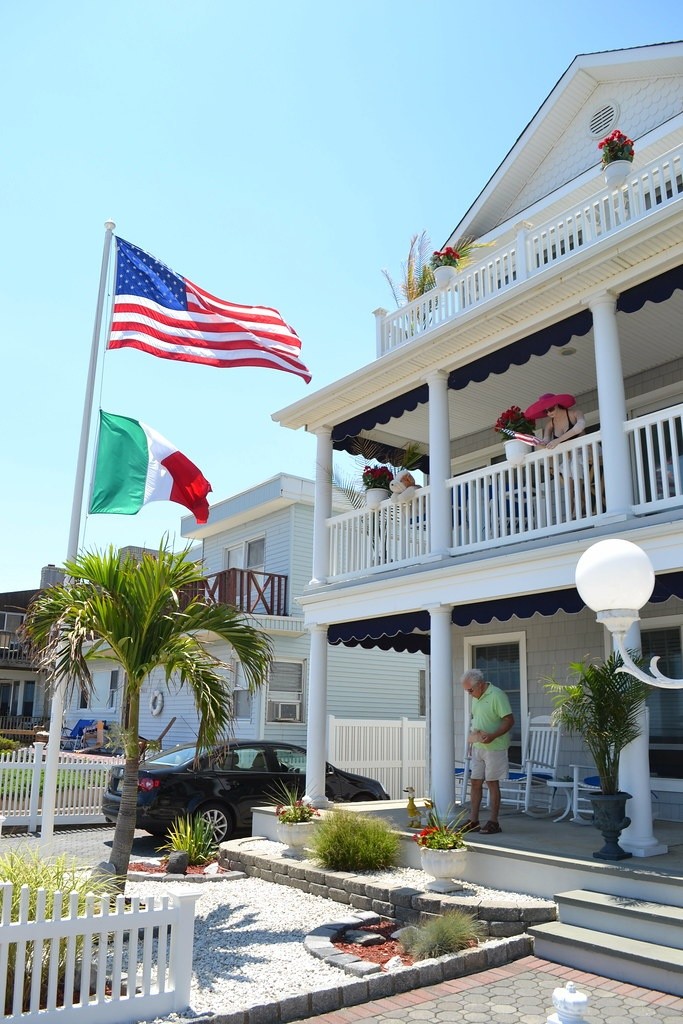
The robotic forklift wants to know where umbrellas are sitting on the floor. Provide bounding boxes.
[499,428,544,446]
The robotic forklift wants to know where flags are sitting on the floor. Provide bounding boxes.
[107,236,312,386]
[88,410,213,524]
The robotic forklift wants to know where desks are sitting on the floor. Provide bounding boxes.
[547,779,583,822]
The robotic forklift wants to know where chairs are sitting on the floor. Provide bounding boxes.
[454,710,615,826]
[60,719,95,751]
[410,483,536,540]
[249,753,266,771]
[35,721,51,742]
[223,752,239,770]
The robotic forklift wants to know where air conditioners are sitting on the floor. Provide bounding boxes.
[274,701,299,721]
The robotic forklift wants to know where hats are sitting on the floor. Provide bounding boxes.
[523,393,578,420]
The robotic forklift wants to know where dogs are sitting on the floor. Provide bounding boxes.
[403,786,429,828]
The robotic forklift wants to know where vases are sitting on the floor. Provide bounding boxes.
[504,439,532,460]
[277,821,314,856]
[366,488,388,509]
[433,265,458,286]
[421,846,469,893]
[603,160,633,187]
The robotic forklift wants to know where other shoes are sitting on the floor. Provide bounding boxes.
[476,818,501,835]
[454,819,479,834]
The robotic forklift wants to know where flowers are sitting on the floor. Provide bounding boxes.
[413,792,482,851]
[495,405,535,440]
[363,465,394,491]
[260,777,327,823]
[598,130,634,169]
[430,246,459,269]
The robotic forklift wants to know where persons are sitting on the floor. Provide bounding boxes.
[460,668,514,834]
[524,393,586,519]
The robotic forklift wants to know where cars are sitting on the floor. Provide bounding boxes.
[101,738,393,852]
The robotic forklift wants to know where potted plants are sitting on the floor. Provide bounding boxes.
[537,648,656,861]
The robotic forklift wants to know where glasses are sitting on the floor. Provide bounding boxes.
[465,683,481,694]
[541,403,557,415]
